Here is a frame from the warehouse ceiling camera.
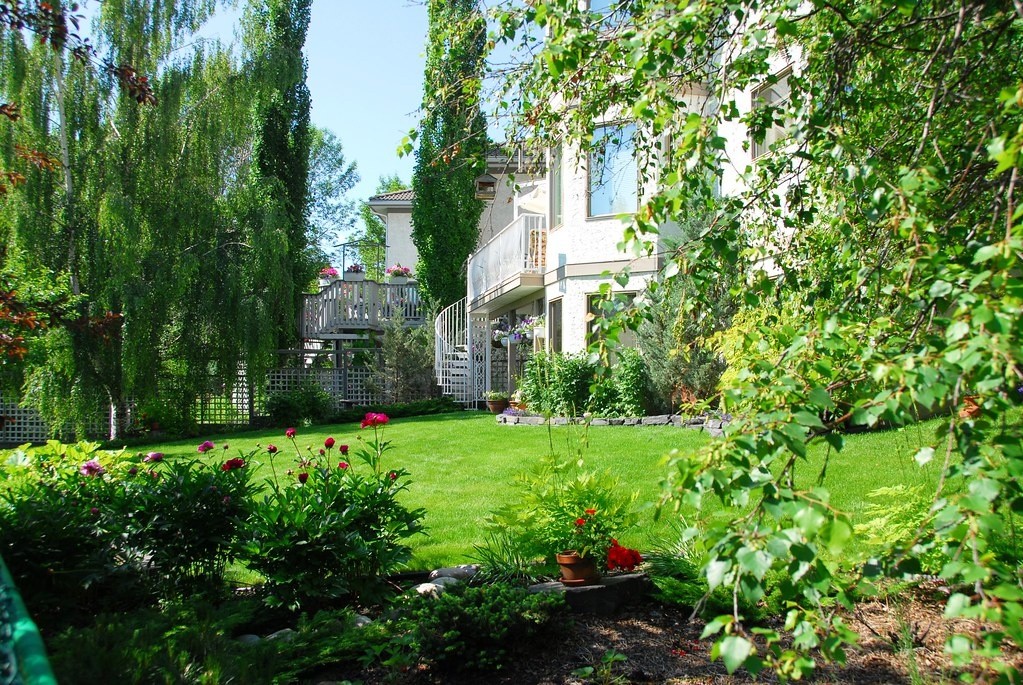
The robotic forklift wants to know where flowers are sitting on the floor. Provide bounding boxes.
[492,313,547,342]
[318,266,338,279]
[556,508,612,559]
[483,390,508,400]
[347,264,364,273]
[386,261,412,278]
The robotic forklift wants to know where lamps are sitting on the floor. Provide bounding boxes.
[473,170,500,201]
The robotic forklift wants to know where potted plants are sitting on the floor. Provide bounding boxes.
[509,394,527,410]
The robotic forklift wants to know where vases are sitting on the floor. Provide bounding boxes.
[343,272,365,282]
[501,338,508,347]
[535,327,545,338]
[320,278,336,285]
[388,276,407,284]
[556,549,598,586]
[487,400,507,413]
[508,334,523,343]
[525,332,535,339]
